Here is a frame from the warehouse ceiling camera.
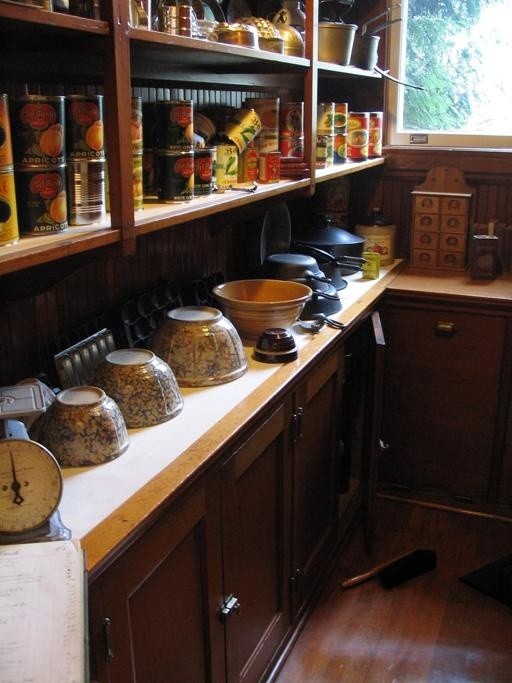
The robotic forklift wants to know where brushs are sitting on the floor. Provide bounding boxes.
[340,548,437,591]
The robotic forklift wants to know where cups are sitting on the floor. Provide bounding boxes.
[361,251,380,279]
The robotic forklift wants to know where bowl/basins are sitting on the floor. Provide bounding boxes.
[96,349,184,427]
[149,306,248,386]
[251,330,298,364]
[214,280,313,340]
[44,386,131,464]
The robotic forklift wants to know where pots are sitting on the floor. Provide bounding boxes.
[318,8,428,93]
[232,202,371,329]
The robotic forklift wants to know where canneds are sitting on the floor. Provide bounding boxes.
[0,93,384,247]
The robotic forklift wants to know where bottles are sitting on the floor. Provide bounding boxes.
[370,207,385,226]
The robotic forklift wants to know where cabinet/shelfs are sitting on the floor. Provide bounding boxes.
[88,454,222,681]
[0,0,388,276]
[343,294,387,555]
[374,288,511,522]
[225,346,342,682]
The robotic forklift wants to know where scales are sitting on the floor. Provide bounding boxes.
[0,383,71,545]
